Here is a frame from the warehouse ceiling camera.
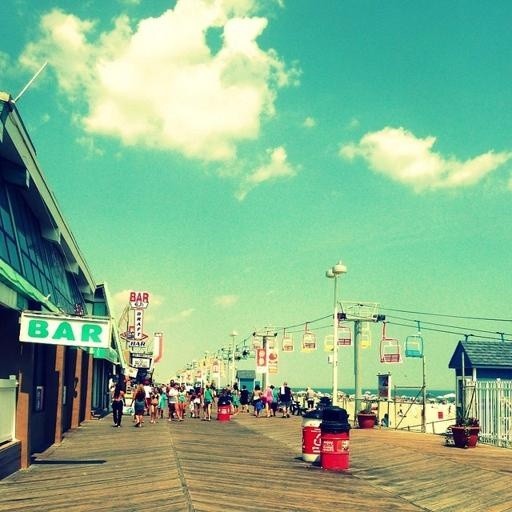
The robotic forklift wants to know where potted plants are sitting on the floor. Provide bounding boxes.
[450,402,480,448]
[357,402,376,429]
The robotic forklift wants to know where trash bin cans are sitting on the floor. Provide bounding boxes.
[218,397,230,421]
[439,412,443,419]
[302,407,350,470]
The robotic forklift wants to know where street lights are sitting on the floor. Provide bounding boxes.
[325,260,347,406]
[230,329,238,388]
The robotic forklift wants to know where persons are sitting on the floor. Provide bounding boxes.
[305,386,318,409]
[108,374,295,430]
[318,391,456,405]
[380,414,388,427]
